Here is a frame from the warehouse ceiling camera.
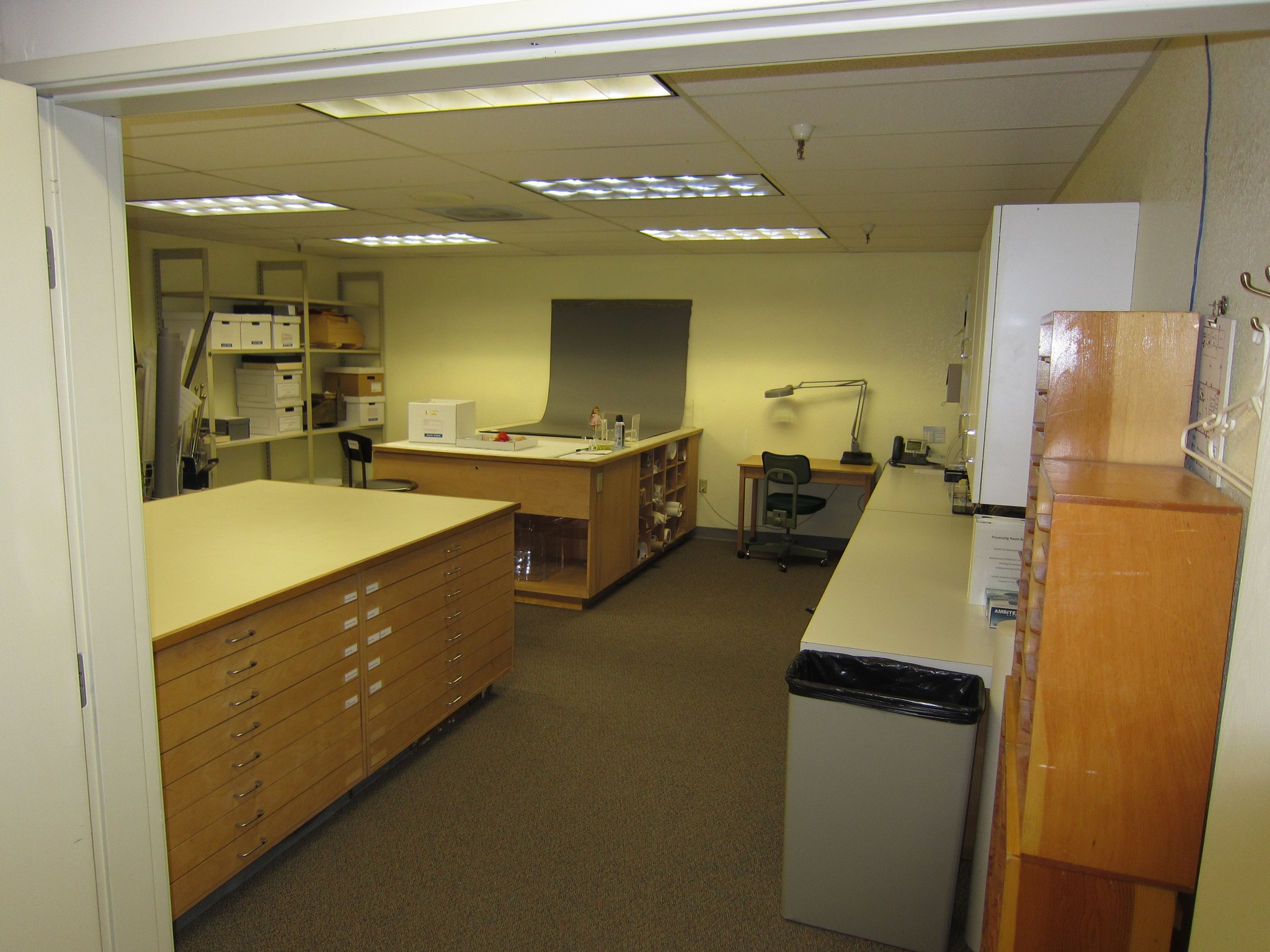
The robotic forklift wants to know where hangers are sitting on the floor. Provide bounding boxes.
[1180,322,1270,499]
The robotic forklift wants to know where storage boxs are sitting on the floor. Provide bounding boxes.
[945,364,963,403]
[408,398,476,447]
[162,309,388,435]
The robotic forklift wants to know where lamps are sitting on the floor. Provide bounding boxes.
[764,377,872,466]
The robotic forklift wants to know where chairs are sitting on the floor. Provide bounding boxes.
[338,431,420,492]
[744,451,830,572]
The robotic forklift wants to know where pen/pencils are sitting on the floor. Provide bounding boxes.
[576,448,589,451]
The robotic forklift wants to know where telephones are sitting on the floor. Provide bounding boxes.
[889,436,931,468]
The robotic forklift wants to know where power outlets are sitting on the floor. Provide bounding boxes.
[699,479,707,493]
[597,472,603,493]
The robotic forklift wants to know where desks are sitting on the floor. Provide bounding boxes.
[800,451,1015,950]
[371,419,704,613]
[143,477,523,927]
[736,454,880,560]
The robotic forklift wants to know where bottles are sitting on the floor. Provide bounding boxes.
[614,415,625,451]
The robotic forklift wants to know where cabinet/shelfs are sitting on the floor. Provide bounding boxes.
[980,308,1247,950]
[956,202,1142,507]
[151,247,386,494]
